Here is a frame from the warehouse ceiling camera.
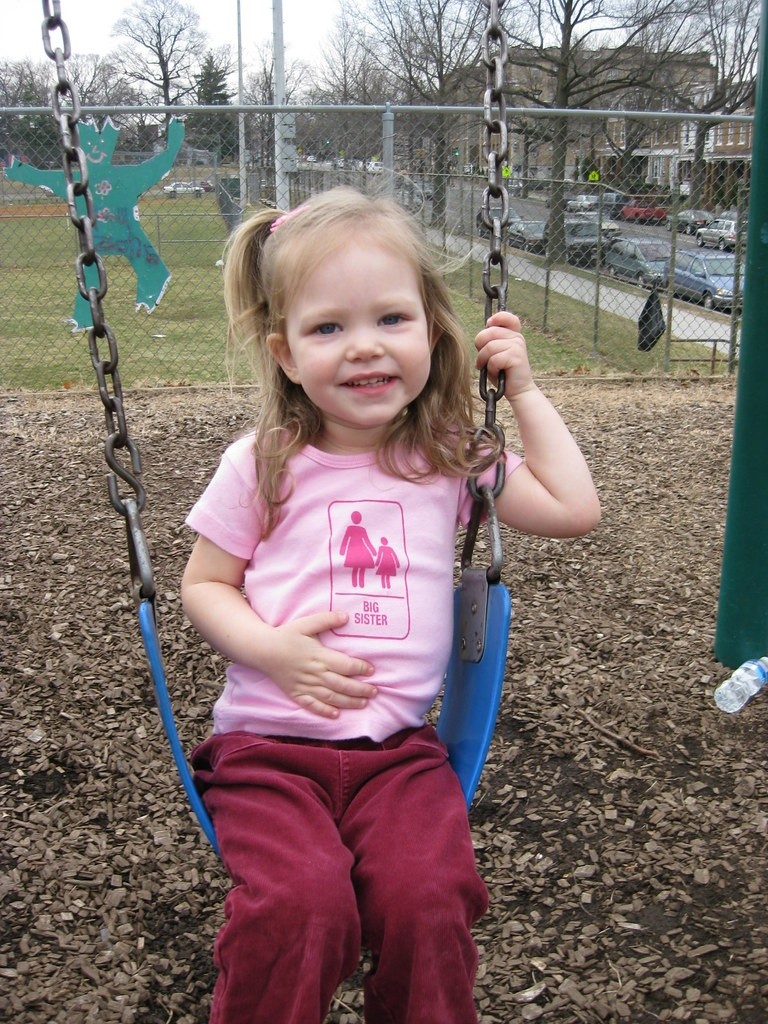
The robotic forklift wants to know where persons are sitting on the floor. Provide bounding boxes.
[180,185,602,1024]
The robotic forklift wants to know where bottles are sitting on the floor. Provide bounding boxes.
[713,657,768,714]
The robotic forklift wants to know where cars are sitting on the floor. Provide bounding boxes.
[665,210,748,251]
[476,203,621,264]
[664,250,746,309]
[545,193,666,221]
[606,237,679,288]
[296,153,317,163]
[506,177,543,198]
[163,180,216,198]
[331,158,383,174]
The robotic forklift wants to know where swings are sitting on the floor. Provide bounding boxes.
[36,0,512,876]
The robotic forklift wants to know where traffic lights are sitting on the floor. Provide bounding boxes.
[455,147,460,156]
[326,138,330,144]
